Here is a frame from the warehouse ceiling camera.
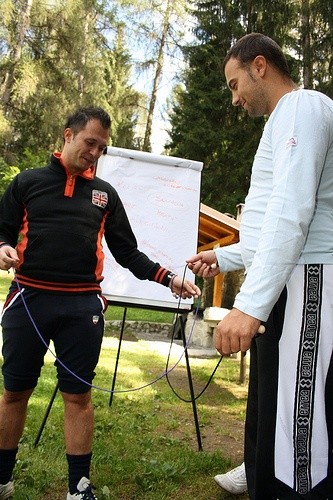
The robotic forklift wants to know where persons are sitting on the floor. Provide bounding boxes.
[0,105,201,500]
[186,33,333,500]
[171,275,177,293]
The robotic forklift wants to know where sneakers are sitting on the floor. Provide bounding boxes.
[213,461,248,495]
[0,481,15,500]
[66,478,98,500]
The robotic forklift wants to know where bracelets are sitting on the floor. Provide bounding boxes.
[0,243,8,246]
